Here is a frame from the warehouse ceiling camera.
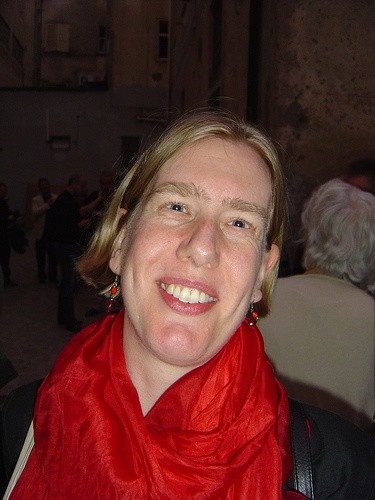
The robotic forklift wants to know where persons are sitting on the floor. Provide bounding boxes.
[257,179,375,423]
[3,175,86,331]
[2,111,375,500]
